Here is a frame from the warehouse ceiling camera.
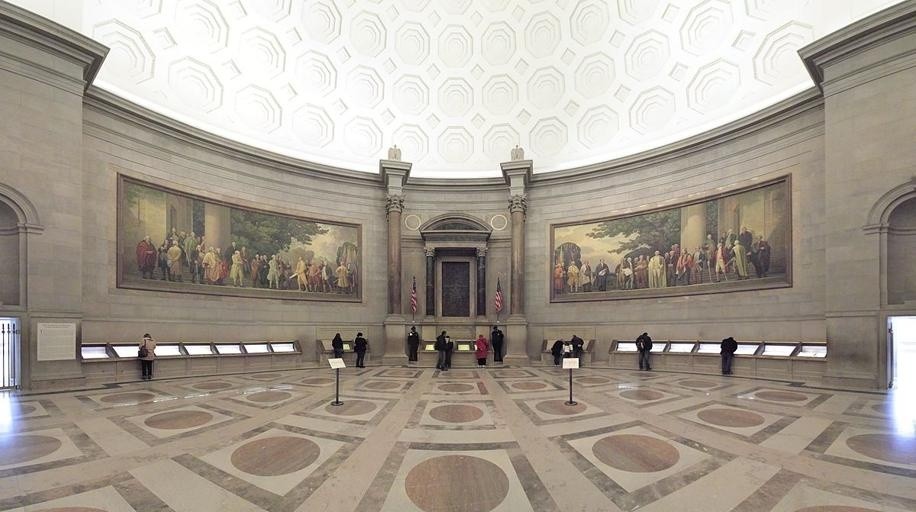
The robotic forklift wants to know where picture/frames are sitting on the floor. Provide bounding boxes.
[114,168,364,305]
[547,172,795,305]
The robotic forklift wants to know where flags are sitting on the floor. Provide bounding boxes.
[495,277,504,312]
[407,279,418,313]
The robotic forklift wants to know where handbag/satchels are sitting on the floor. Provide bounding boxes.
[138,339,148,357]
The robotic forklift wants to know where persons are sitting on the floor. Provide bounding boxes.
[635,331,653,371]
[446,336,453,369]
[491,326,504,362]
[136,333,157,380]
[550,340,563,365]
[331,332,344,358]
[435,329,448,371]
[135,226,358,297]
[407,326,420,362]
[475,334,489,368]
[353,331,368,369]
[719,336,738,375]
[572,334,584,368]
[552,225,771,294]
